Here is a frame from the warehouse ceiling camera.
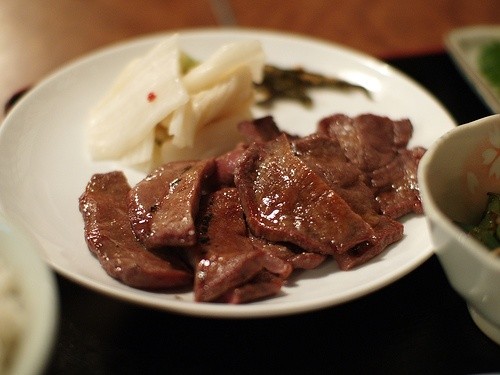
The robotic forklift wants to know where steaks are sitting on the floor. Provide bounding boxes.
[78,113,427,305]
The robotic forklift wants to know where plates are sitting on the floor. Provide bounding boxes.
[1,212,59,374]
[0,27,460,317]
[441,26,500,118]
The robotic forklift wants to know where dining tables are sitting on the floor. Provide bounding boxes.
[1,41,500,374]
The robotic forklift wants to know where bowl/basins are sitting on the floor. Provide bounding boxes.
[418,115,500,346]
